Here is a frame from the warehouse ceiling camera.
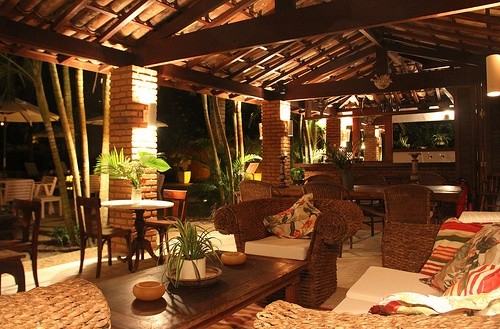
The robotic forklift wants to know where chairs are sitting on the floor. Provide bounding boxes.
[239,168,437,258]
[1,175,189,290]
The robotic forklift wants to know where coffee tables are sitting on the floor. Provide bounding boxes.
[93,249,301,329]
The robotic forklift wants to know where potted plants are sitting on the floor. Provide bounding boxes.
[163,217,225,288]
[370,74,393,89]
[172,158,193,184]
[289,167,305,184]
[94,146,172,203]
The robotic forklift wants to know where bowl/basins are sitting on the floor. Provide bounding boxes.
[133,281,165,301]
[221,252,247,265]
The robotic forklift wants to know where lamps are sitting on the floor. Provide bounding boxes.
[258,119,294,139]
[142,103,158,130]
[486,53,500,97]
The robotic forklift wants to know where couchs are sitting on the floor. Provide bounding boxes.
[216,198,363,305]
[253,221,500,329]
[1,278,112,329]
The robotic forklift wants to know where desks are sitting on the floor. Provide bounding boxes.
[99,198,173,271]
[353,183,462,236]
[273,186,303,198]
[35,195,62,219]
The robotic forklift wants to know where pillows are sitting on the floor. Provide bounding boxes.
[416,217,500,295]
